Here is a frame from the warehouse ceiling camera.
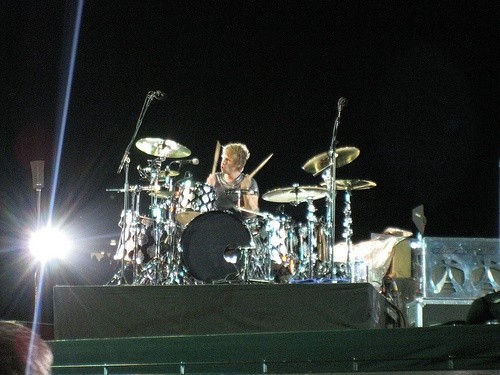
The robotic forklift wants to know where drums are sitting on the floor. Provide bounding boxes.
[243,213,297,268]
[172,210,253,283]
[149,177,180,199]
[226,189,261,215]
[292,222,330,275]
[116,213,170,267]
[172,180,216,223]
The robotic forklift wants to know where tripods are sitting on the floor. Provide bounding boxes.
[106,99,182,289]
[273,108,359,285]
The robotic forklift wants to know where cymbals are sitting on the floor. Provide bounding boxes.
[262,187,327,201]
[140,166,180,177]
[301,147,360,173]
[317,178,377,189]
[135,138,192,158]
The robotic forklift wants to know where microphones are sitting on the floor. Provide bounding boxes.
[149,91,167,100]
[173,158,199,165]
[338,98,348,107]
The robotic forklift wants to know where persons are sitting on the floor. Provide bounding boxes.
[1,322,55,375]
[199,141,260,216]
